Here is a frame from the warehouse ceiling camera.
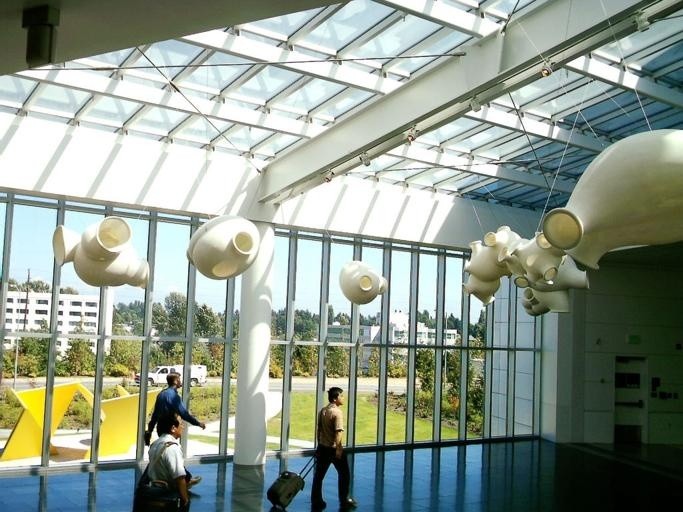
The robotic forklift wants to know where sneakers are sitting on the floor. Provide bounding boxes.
[340,497,358,509]
[312,502,326,512]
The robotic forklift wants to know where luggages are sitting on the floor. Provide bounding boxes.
[267,451,317,511]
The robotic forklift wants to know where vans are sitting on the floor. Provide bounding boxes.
[134,363,207,387]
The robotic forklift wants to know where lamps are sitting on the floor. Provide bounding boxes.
[324,11,650,181]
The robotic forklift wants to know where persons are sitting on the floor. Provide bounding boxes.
[309,386,360,511]
[141,371,205,491]
[143,404,192,512]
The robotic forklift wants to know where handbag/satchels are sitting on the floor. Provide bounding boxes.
[133,480,190,512]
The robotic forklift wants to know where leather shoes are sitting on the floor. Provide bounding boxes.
[187,476,201,488]
